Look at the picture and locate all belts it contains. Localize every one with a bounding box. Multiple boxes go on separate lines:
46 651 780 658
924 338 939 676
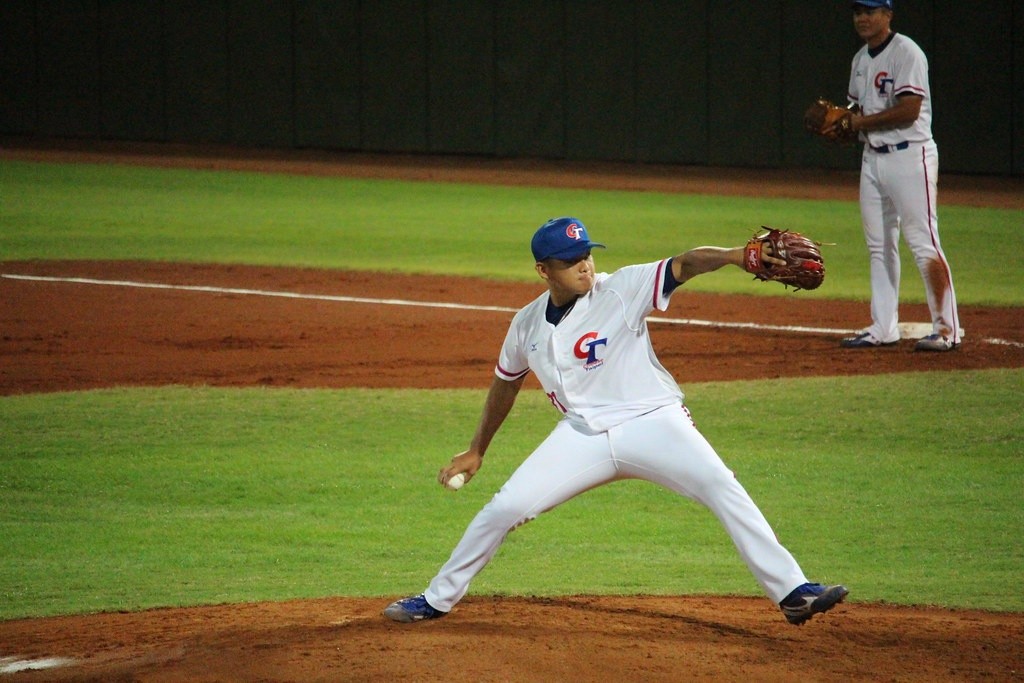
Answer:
869 141 908 154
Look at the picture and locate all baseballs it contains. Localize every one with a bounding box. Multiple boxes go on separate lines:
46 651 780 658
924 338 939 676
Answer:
444 472 465 490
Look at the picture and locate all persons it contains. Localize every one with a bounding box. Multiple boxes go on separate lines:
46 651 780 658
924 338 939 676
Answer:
383 217 849 626
803 0 962 352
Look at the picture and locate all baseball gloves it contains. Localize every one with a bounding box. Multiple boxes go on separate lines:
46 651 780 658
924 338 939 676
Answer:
803 95 859 150
742 222 839 293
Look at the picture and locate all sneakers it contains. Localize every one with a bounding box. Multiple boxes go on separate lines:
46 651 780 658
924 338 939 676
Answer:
780 581 848 626
917 333 960 351
841 332 897 347
385 594 447 623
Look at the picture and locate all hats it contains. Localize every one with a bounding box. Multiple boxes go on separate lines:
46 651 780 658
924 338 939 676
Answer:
532 216 606 262
853 0 892 10
